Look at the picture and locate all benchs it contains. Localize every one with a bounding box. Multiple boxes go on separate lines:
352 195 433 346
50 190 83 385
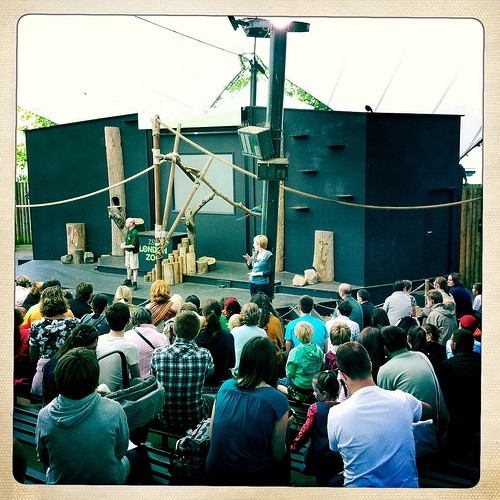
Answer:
12 386 472 488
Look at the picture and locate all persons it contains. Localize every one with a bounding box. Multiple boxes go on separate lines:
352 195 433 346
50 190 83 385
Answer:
206 336 290 486
242 234 275 302
34 347 132 485
276 273 482 460
120 218 140 291
14 273 285 435
327 341 432 488
290 370 345 475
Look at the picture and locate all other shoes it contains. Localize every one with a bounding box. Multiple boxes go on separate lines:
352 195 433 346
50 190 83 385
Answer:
132 283 137 291
120 279 132 287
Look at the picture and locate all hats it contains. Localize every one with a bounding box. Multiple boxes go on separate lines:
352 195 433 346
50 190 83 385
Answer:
458 315 478 333
223 297 241 317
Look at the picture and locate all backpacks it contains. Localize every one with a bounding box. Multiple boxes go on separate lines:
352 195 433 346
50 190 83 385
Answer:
167 416 212 485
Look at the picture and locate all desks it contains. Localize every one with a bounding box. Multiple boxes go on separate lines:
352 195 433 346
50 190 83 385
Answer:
137 229 187 271
